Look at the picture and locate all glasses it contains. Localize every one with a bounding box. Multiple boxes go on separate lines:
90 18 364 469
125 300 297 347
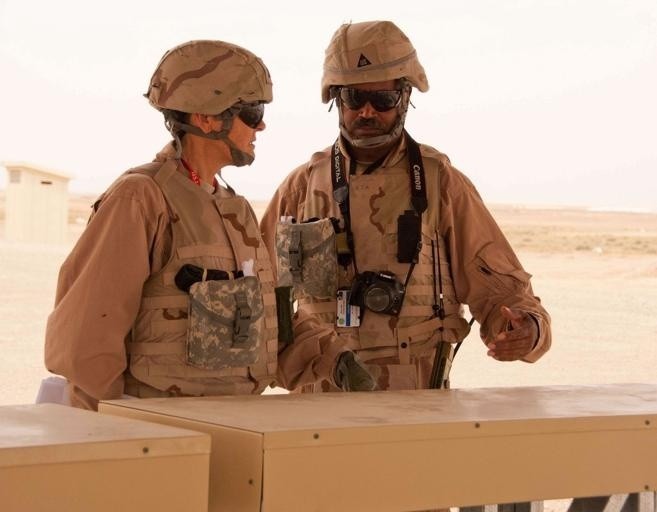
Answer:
340 87 402 111
238 104 264 128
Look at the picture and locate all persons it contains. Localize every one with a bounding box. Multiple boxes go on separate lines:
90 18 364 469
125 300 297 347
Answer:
261 19 552 394
43 40 382 413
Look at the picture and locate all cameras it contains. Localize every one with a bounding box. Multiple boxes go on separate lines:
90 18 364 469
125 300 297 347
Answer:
347 270 406 316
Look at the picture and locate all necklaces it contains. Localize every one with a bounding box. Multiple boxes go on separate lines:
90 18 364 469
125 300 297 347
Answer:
182 158 221 195
342 137 398 182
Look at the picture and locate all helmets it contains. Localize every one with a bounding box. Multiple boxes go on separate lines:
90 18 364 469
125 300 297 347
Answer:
322 22 428 104
147 40 272 115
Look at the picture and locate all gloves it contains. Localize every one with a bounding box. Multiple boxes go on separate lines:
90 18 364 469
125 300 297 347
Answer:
337 351 377 391
